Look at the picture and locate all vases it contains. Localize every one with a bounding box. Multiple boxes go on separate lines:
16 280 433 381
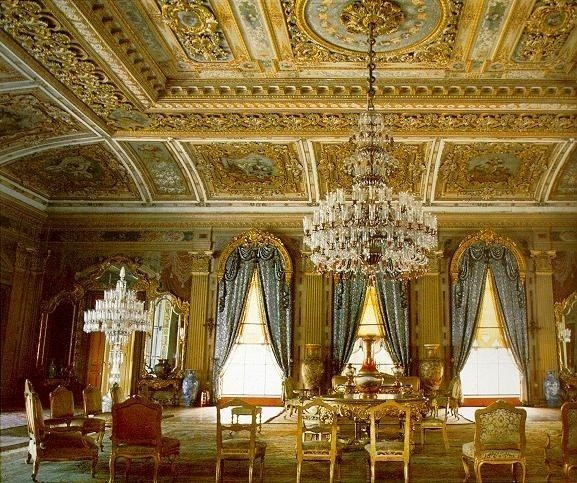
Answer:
163 358 172 375
301 342 324 395
419 343 444 397
46 358 58 381
182 368 199 408
543 371 561 408
154 359 168 379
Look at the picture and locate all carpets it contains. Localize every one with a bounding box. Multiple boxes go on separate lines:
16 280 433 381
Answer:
0 419 565 483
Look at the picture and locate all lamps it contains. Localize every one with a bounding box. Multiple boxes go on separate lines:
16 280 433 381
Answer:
83 267 155 410
299 0 440 282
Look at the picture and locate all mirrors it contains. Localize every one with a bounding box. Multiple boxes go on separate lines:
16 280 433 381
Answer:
139 292 190 389
36 291 82 385
554 291 577 387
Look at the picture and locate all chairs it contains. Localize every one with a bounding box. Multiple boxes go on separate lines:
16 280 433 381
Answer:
419 400 451 451
362 399 412 483
213 397 268 482
543 401 576 482
295 398 338 482
460 398 528 483
282 377 309 418
21 379 180 483
